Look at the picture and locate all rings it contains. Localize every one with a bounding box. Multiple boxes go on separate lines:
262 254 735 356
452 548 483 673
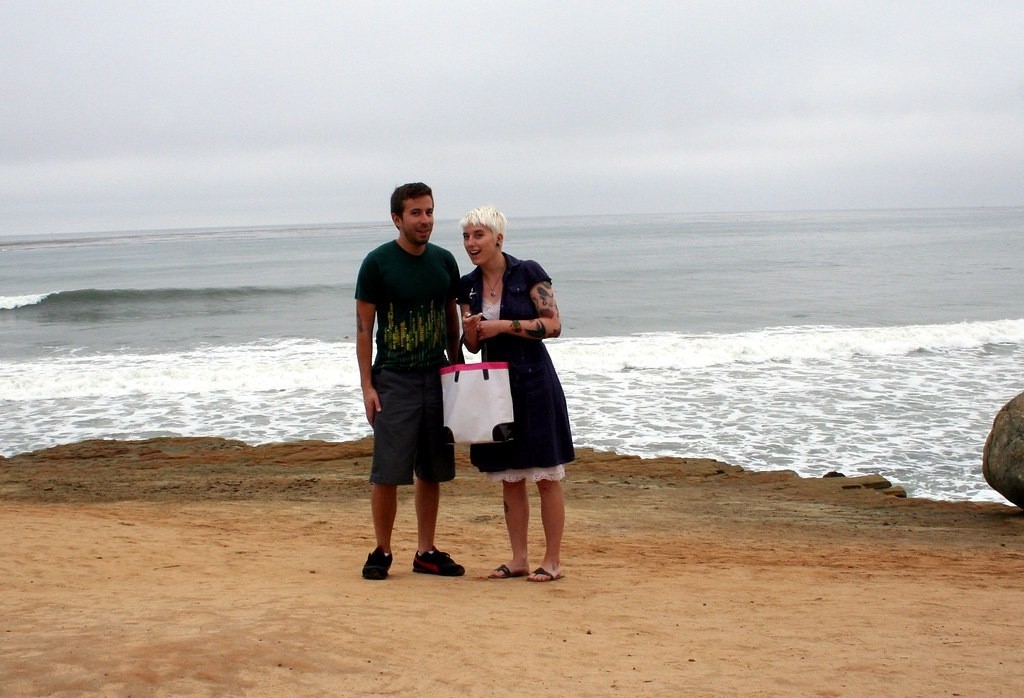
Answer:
476 327 481 331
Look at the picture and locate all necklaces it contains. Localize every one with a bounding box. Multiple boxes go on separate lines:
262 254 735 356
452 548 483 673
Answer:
481 269 504 297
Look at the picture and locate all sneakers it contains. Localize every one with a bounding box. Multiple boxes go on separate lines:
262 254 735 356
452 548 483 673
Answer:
362 545 392 579
413 546 465 576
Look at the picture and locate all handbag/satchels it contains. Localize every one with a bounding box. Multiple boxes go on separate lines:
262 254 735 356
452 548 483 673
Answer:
440 331 516 443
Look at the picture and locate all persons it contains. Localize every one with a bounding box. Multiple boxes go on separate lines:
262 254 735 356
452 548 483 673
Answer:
353 182 465 580
458 206 575 582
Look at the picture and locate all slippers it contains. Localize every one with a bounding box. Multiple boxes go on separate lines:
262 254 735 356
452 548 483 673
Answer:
528 568 565 581
488 564 530 579
